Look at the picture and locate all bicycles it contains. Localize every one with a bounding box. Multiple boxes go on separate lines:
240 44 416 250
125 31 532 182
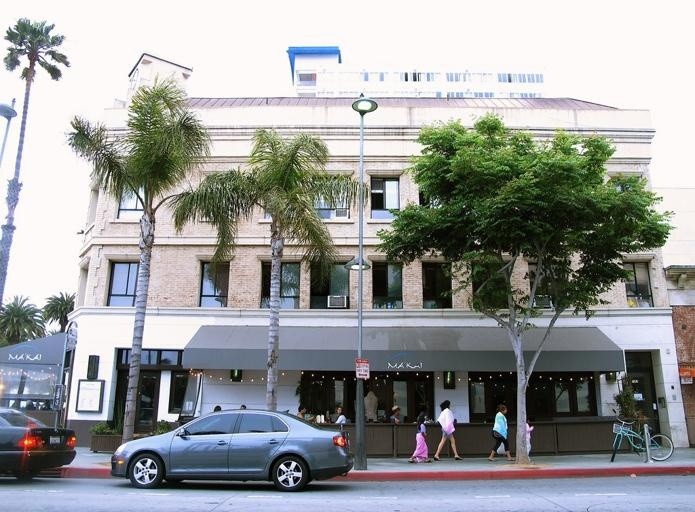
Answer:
611 408 674 462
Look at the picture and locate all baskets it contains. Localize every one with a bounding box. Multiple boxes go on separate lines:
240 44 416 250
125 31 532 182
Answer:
613 422 630 437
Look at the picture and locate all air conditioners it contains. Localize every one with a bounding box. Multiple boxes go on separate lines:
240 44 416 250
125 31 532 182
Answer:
327 295 349 309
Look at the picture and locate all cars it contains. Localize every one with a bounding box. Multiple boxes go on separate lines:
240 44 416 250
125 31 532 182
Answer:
0 407 76 482
111 408 353 492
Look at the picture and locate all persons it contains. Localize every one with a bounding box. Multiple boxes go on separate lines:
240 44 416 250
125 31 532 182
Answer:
214 406 221 411
432 399 463 460
488 404 515 461
239 404 246 409
297 405 316 422
525 416 536 457
334 405 346 423
389 405 401 423
408 411 431 463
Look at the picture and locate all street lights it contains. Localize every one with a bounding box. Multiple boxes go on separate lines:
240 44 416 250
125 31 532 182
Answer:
346 93 378 470
